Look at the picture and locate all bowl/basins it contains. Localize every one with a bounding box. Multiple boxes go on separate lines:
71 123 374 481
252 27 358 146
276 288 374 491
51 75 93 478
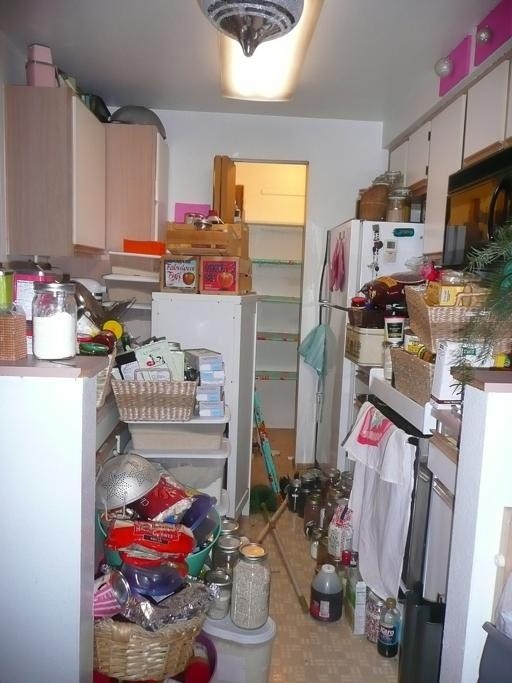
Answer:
97 505 223 580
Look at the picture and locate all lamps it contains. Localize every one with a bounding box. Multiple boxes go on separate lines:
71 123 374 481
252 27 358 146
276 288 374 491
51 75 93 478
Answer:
197 0 306 59
215 1 323 103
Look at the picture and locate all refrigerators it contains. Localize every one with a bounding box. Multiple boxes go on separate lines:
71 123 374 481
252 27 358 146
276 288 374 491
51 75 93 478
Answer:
313 221 424 486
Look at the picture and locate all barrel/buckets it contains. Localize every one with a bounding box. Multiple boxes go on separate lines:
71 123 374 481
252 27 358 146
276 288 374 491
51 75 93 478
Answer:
309 564 344 622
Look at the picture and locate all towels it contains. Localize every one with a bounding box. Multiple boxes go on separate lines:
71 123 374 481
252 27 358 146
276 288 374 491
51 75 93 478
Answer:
298 324 325 374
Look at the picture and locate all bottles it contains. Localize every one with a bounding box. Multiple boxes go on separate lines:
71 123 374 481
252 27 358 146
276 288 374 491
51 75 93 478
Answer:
168 341 181 352
93 328 117 353
376 597 399 657
342 548 361 607
181 640 210 683
383 303 435 363
0 263 14 313
207 517 272 630
373 168 410 222
284 467 354 558
30 280 77 361
350 296 366 308
184 208 221 230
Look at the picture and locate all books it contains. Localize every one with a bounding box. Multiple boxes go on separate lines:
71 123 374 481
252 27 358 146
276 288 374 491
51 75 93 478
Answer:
115 339 186 383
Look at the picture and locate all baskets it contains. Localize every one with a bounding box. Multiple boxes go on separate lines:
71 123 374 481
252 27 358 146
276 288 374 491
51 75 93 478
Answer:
110 371 202 423
95 348 120 409
92 613 207 682
389 344 437 408
403 276 512 356
0 313 28 362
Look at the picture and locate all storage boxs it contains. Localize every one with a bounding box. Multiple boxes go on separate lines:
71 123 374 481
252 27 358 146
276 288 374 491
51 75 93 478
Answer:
131 436 230 506
120 404 230 452
343 324 386 366
101 252 163 344
202 608 278 683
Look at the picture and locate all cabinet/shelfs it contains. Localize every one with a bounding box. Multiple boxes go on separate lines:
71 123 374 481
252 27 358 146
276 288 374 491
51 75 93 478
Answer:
389 47 512 194
250 223 305 431
1 84 106 257
448 168 506 246
0 338 137 682
102 123 171 256
